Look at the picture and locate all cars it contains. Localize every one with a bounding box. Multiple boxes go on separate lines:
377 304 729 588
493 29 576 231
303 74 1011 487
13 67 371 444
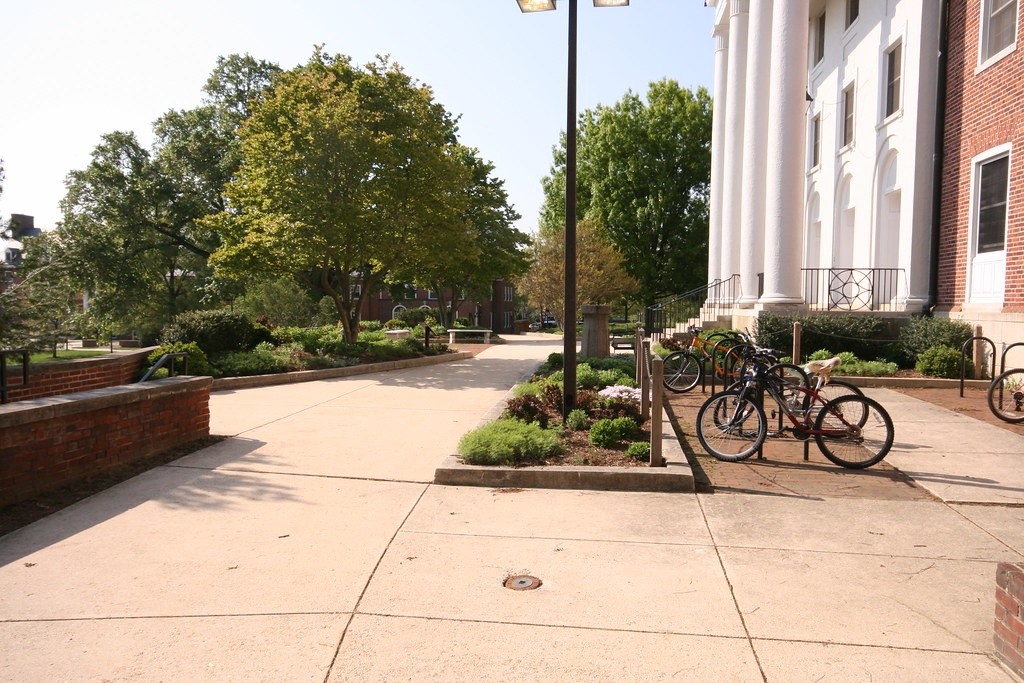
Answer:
529 315 558 332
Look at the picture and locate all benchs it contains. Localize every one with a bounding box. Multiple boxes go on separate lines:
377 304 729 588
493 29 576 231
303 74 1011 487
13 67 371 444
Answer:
385 330 410 339
448 328 493 343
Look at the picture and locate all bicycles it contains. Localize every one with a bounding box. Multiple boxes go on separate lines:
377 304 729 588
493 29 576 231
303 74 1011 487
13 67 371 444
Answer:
695 345 895 471
986 365 1024 424
662 323 870 438
